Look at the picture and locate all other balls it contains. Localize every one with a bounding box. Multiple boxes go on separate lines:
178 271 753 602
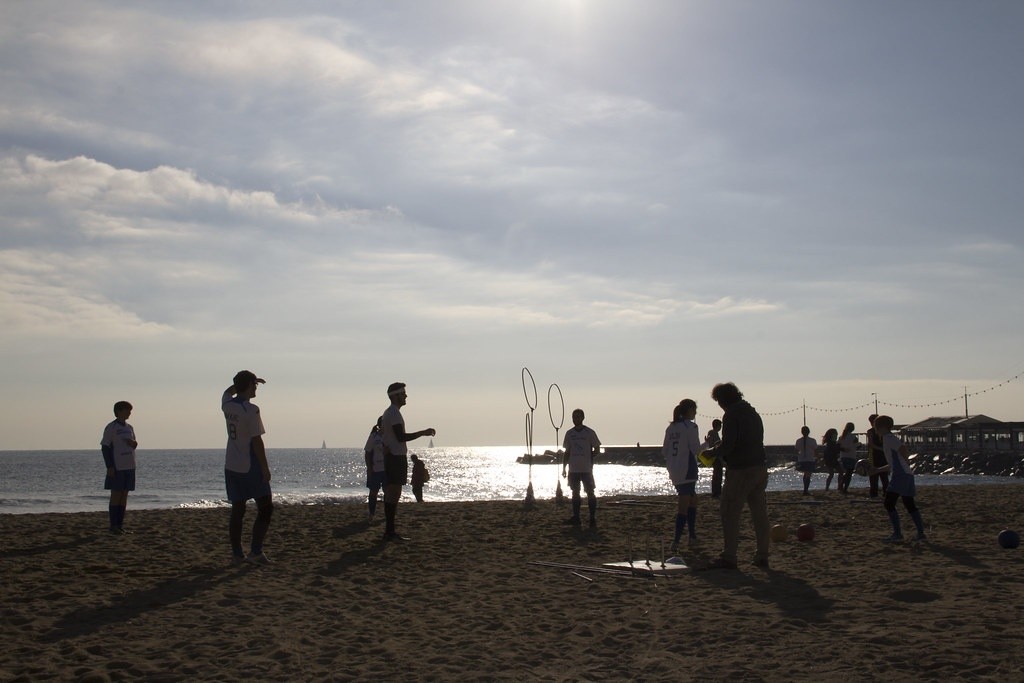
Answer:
998 530 1021 549
797 523 814 541
855 459 871 476
769 522 788 541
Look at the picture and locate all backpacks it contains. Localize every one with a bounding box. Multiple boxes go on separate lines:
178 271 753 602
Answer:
423 468 430 483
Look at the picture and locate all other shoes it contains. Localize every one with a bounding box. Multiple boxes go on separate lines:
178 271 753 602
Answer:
232 551 273 571
383 532 411 543
911 533 928 541
882 533 905 543
671 541 685 550
589 517 596 529
569 516 581 525
109 527 133 535
688 537 705 546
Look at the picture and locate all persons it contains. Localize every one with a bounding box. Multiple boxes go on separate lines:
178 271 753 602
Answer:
822 428 849 490
562 409 602 526
866 413 890 496
410 455 430 503
840 422 860 494
221 371 277 566
706 419 723 498
99 401 138 535
382 382 436 543
363 416 383 525
703 383 771 565
871 415 929 539
661 399 713 551
794 426 818 495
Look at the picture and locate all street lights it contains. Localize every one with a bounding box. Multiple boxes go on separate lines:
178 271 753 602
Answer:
871 392 878 415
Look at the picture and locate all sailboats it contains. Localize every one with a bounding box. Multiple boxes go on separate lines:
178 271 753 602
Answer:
428 439 434 448
321 440 326 449
